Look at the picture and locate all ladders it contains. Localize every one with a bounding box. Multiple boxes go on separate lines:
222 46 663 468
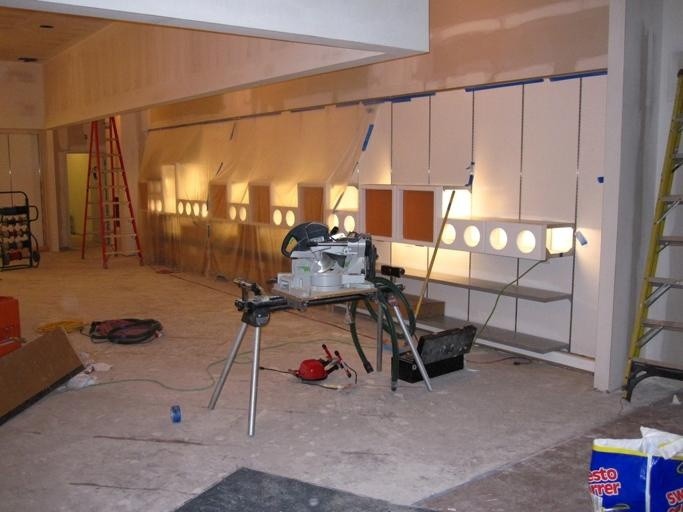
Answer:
621 68 683 403
81 114 145 270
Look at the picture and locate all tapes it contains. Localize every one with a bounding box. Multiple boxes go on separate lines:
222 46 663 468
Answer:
171 405 180 422
